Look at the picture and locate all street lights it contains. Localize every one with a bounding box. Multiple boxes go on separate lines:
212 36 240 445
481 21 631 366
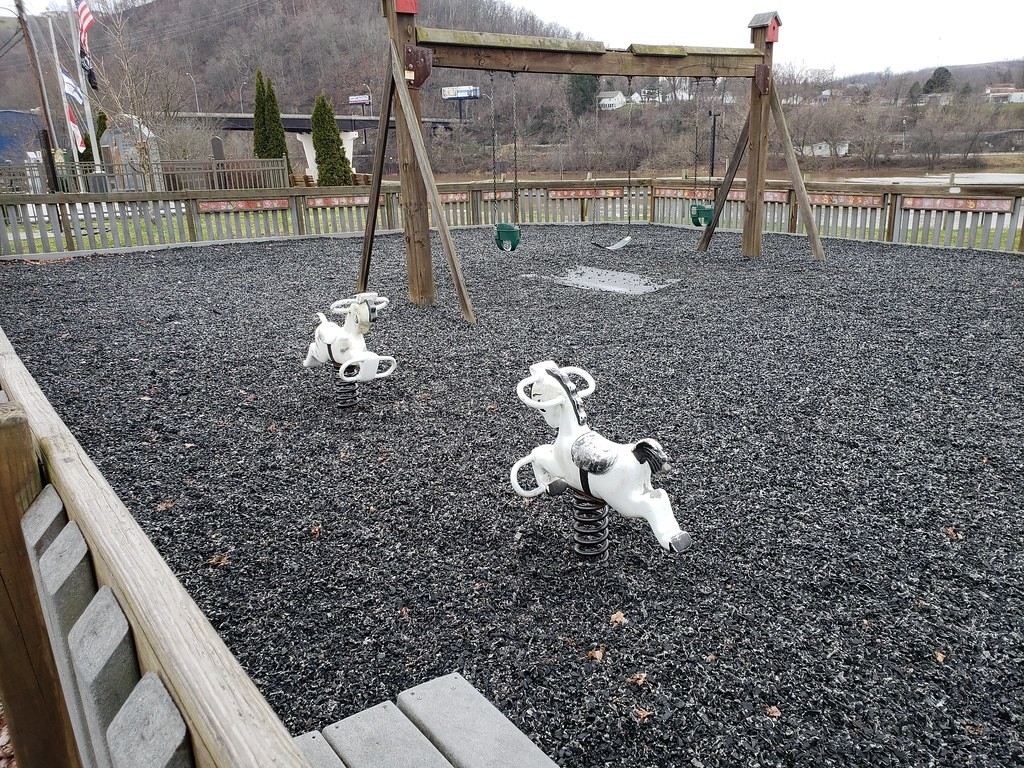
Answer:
363 84 372 116
185 73 199 112
240 82 247 114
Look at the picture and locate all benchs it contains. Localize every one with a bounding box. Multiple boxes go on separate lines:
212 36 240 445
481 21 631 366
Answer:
290 671 562 766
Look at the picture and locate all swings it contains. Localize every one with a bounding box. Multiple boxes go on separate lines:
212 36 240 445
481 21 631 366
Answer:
690 79 716 228
489 73 522 252
590 76 633 251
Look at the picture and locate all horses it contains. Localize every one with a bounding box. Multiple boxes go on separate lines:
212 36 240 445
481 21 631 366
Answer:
509 360 694 555
302 292 397 381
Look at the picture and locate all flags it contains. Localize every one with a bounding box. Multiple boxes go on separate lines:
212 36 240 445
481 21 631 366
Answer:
74 0 95 52
61 68 84 106
79 34 98 92
68 104 86 153
